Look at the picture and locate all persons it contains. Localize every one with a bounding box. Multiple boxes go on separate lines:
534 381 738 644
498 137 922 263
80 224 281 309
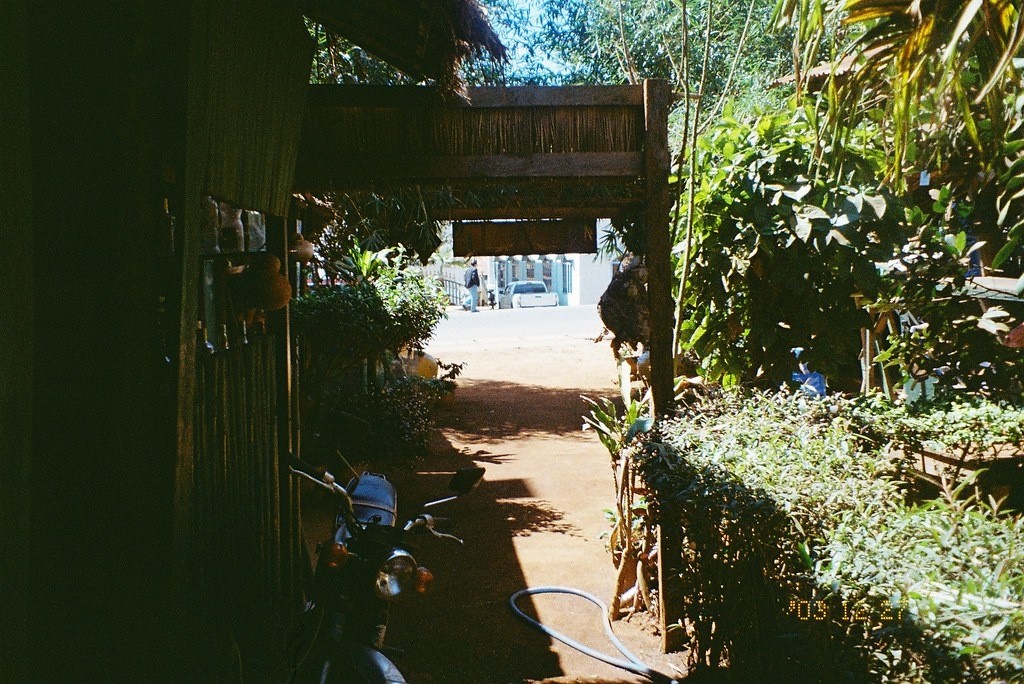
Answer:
462 259 480 312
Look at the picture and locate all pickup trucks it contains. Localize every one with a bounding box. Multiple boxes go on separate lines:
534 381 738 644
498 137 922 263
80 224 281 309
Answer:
498 281 558 308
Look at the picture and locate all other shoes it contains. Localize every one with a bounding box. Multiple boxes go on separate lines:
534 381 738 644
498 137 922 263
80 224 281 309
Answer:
461 304 468 311
471 308 479 312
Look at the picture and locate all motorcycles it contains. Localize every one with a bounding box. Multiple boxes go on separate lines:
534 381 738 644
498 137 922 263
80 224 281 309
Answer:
283 438 485 684
